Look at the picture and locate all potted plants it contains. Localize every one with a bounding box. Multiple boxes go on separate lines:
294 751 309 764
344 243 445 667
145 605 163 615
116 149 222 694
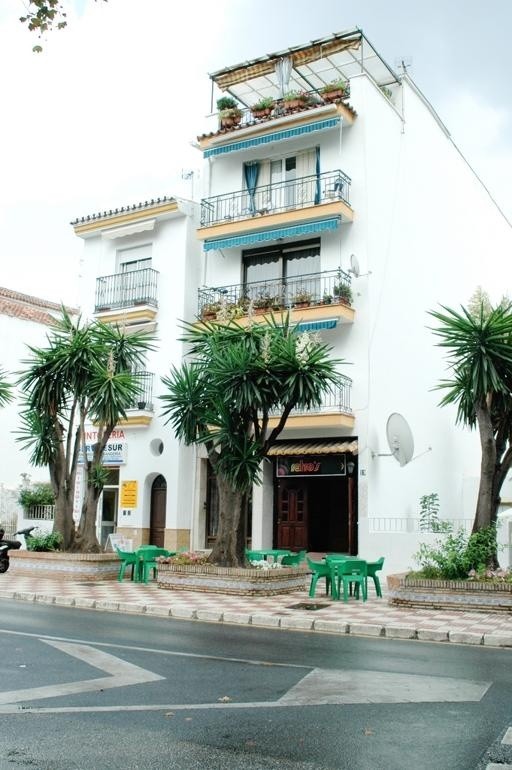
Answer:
217 78 348 128
200 281 351 320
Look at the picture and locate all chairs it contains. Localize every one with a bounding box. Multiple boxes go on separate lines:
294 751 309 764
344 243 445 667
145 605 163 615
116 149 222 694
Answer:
245 547 307 566
115 544 187 584
305 555 385 603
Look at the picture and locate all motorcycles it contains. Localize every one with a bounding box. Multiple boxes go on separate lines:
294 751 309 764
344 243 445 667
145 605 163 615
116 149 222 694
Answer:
0 526 39 573
